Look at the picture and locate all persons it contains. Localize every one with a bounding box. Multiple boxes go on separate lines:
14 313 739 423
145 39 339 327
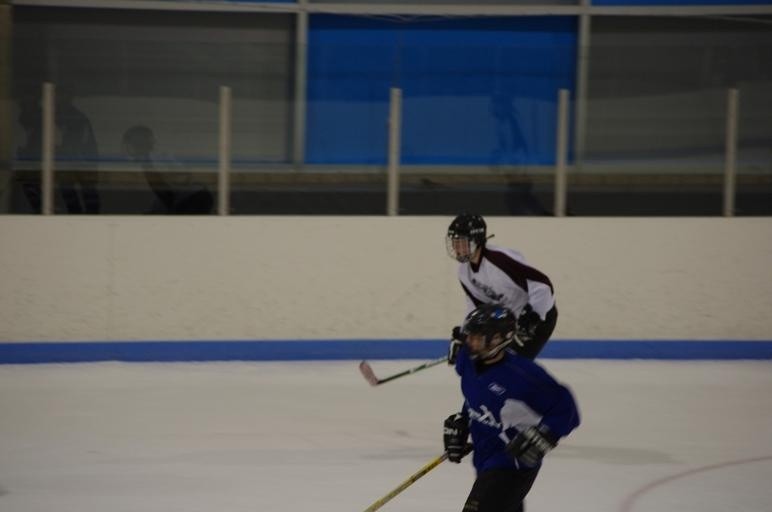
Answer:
445 211 558 366
442 304 581 512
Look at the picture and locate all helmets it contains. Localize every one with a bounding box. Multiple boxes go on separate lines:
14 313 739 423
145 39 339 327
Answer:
445 212 486 262
461 305 515 362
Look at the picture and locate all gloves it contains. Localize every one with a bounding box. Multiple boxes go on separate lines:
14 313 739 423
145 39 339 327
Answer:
443 412 467 463
509 428 552 471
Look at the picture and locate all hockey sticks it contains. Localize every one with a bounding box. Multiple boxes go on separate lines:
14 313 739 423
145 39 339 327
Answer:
360 357 449 385
